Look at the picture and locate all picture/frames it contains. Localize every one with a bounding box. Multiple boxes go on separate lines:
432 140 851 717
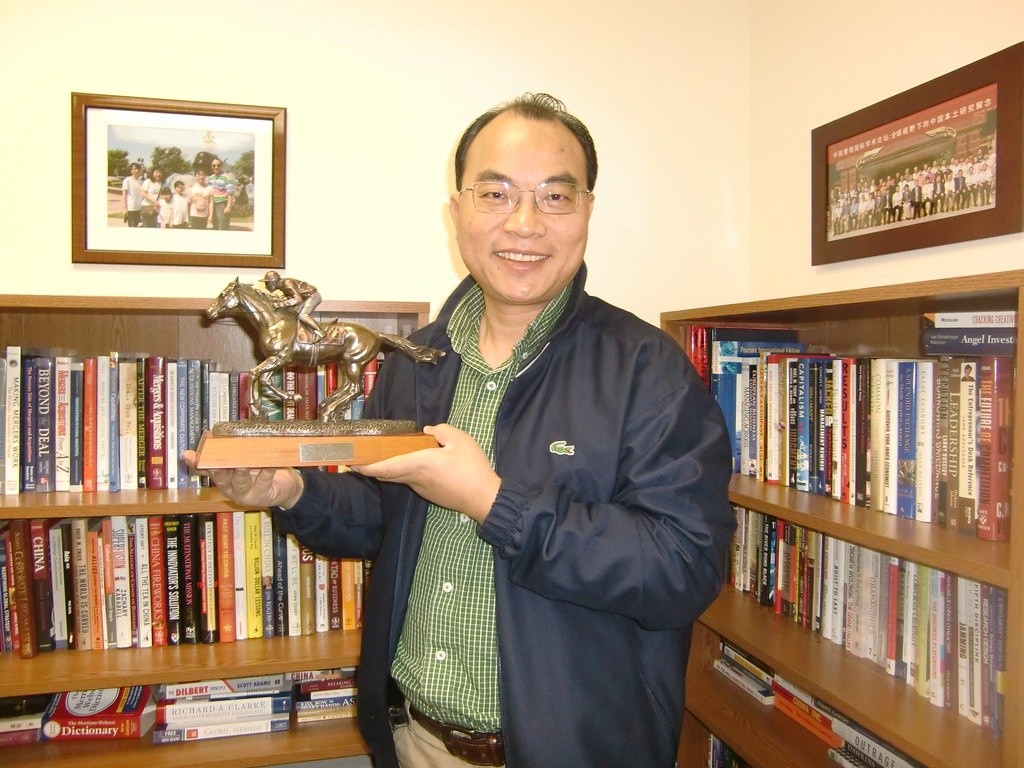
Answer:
71 91 288 268
810 40 1024 266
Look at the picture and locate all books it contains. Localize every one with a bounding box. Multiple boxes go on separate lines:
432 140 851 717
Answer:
707 734 750 768
0 510 374 659
714 640 924 768
728 505 1007 739
0 346 385 492
685 311 1019 541
0 667 358 747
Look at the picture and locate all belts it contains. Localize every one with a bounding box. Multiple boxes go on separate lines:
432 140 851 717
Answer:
402 699 505 767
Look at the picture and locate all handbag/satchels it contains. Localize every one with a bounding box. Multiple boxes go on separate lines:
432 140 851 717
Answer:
153 206 160 214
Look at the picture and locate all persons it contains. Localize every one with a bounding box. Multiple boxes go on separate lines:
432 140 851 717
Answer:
961 364 975 382
828 145 997 236
263 576 272 590
122 158 254 230
182 92 737 768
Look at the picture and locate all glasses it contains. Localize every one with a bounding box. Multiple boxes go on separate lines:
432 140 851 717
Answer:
212 165 220 168
459 181 589 214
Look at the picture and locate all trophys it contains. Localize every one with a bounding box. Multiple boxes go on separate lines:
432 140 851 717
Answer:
196 271 446 468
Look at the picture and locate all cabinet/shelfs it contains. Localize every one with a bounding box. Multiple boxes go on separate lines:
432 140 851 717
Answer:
0 295 432 768
658 267 1023 768
